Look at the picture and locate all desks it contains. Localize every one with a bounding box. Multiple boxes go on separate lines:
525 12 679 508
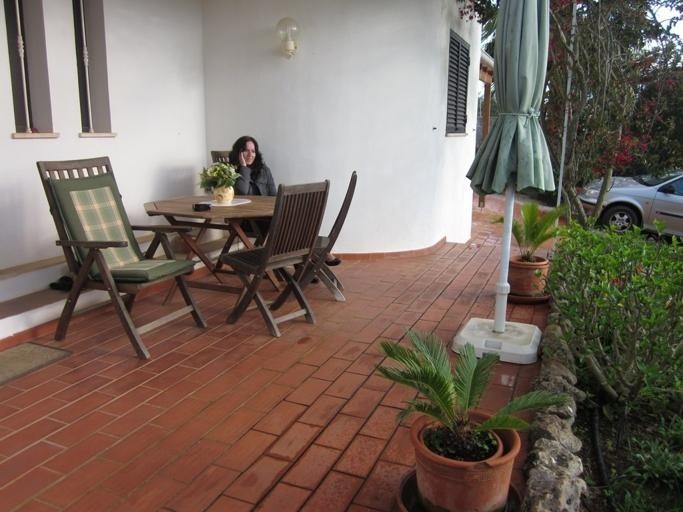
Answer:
143 195 283 313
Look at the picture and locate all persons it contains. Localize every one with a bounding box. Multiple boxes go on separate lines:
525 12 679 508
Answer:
229 136 342 284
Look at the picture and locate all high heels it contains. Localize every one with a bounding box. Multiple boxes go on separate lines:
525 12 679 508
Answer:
292 252 341 284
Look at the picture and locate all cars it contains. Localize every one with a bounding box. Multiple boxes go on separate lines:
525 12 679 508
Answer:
575 169 682 246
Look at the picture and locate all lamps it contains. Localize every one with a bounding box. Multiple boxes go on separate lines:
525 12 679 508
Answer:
276 16 301 58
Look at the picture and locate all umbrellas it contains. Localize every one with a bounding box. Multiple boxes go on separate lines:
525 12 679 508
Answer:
466 0 556 333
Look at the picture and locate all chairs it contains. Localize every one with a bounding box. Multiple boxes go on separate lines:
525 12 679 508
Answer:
36 156 209 359
287 171 357 301
220 179 330 338
211 151 285 281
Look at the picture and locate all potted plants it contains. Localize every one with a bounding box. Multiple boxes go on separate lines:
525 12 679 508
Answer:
196 161 241 202
490 200 568 303
375 326 568 512
